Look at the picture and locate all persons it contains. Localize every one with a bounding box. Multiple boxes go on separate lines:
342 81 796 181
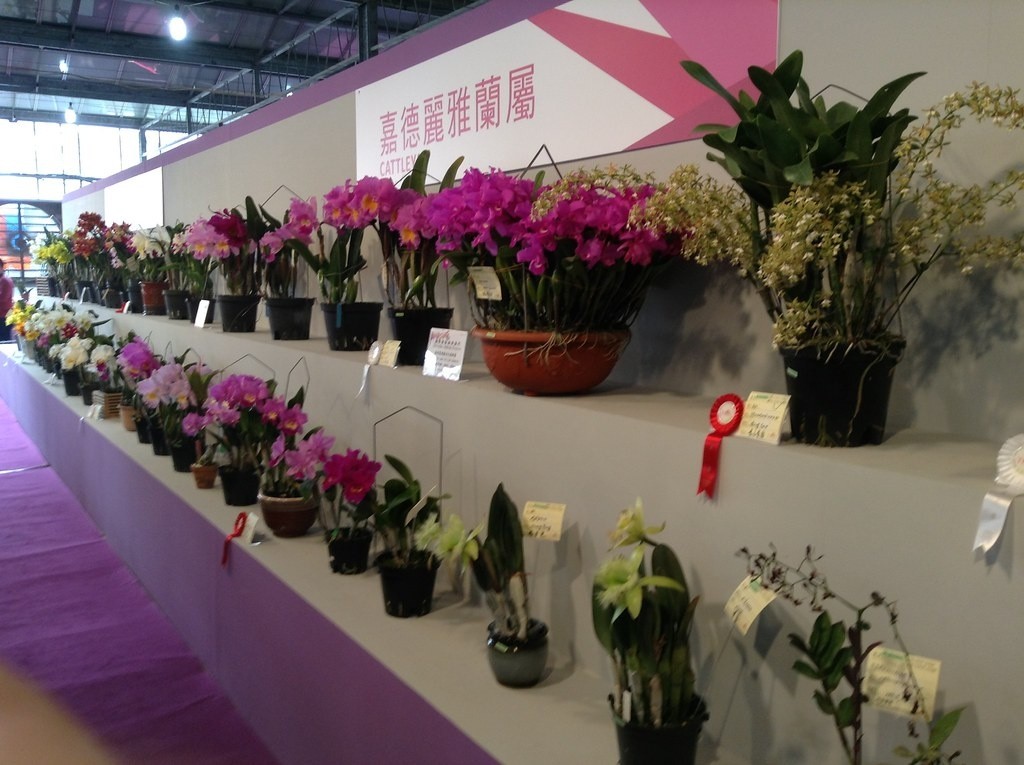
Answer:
0 259 13 341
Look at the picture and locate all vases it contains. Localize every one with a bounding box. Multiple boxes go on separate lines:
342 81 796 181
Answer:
185 298 216 324
15 335 22 351
471 325 632 396
79 382 101 405
327 527 372 574
18 334 27 352
321 302 383 353
138 282 170 316
388 307 454 366
53 361 63 379
98 281 121 306
778 330 906 448
192 463 218 489
56 283 62 297
75 281 93 299
24 339 37 358
116 403 142 431
132 414 161 443
45 359 55 373
149 423 175 456
218 464 264 506
487 618 549 688
218 295 262 333
257 480 319 538
170 441 200 471
376 550 440 618
265 298 316 342
87 287 97 303
36 276 55 296
162 290 190 319
38 351 43 365
62 370 81 395
611 693 709 764
126 287 143 313
92 389 123 419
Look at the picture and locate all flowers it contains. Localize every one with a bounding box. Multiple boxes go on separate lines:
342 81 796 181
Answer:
33 50 1024 449
5 289 967 764
